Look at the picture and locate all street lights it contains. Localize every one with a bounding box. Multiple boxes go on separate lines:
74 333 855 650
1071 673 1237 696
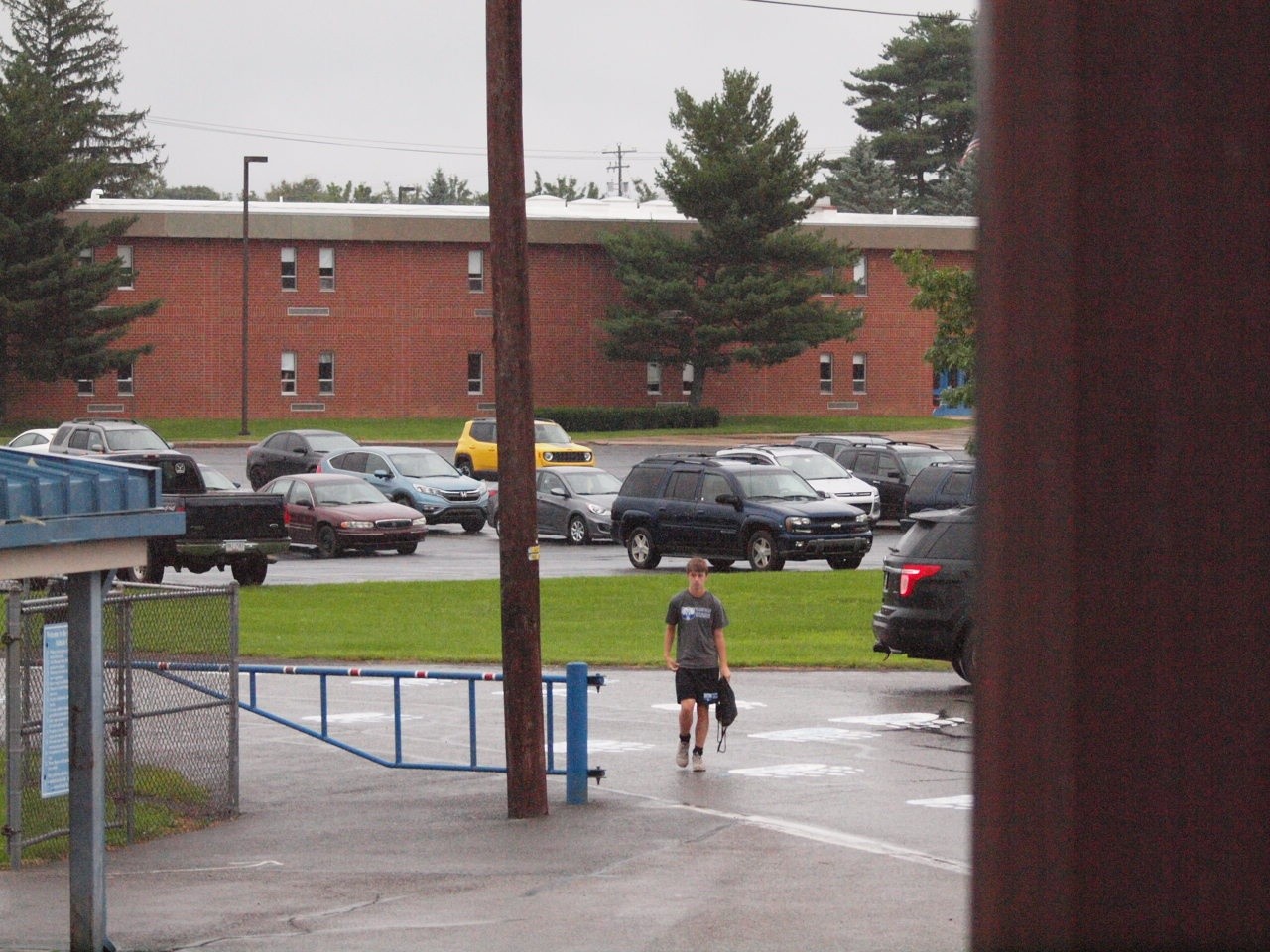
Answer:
240 154 268 435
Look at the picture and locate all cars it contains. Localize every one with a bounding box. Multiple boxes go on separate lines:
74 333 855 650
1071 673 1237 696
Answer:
714 443 882 523
196 463 242 493
484 464 623 546
254 472 429 559
6 427 57 455
245 429 362 493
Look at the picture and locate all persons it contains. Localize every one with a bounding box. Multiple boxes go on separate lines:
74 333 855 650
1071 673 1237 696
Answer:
664 557 731 771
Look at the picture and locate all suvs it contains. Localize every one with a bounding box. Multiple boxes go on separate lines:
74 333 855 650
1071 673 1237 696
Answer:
898 459 977 535
453 415 596 481
833 440 960 533
870 503 978 684
315 445 491 534
609 451 873 573
48 416 175 456
789 433 898 477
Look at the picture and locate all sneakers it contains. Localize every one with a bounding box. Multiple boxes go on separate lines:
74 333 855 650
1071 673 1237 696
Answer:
675 737 690 767
691 751 707 771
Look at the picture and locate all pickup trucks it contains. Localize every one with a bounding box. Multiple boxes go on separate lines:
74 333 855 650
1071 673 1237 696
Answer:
72 449 293 586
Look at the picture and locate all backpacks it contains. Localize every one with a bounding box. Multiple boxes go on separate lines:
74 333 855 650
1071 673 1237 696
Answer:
716 676 738 753
